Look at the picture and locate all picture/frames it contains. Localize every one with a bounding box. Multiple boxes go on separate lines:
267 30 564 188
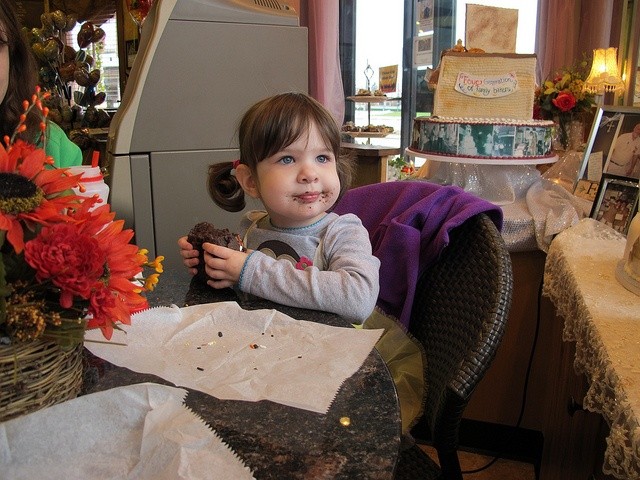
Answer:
571 178 600 204
574 104 640 180
588 173 640 236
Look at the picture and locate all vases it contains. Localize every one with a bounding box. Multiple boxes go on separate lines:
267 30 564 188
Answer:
0 316 163 422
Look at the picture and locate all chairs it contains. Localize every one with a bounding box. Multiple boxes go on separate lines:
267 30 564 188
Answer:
332 179 515 480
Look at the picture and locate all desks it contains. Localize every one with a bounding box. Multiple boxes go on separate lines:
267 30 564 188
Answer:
542 216 640 480
83 275 401 480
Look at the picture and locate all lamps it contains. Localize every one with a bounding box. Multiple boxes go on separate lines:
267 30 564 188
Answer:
581 47 624 105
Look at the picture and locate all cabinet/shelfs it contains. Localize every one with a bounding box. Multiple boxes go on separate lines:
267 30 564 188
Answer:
539 309 603 480
462 252 556 432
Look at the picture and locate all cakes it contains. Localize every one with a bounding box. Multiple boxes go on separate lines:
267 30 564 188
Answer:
410 116 555 159
184 223 246 284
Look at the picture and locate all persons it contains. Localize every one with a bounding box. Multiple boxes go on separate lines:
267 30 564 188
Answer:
0 3 86 224
177 94 381 327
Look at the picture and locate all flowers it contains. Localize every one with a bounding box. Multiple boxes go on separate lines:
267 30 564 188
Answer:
0 85 166 340
535 61 596 147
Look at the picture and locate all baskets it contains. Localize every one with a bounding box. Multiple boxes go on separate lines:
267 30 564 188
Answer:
0 311 84 424
